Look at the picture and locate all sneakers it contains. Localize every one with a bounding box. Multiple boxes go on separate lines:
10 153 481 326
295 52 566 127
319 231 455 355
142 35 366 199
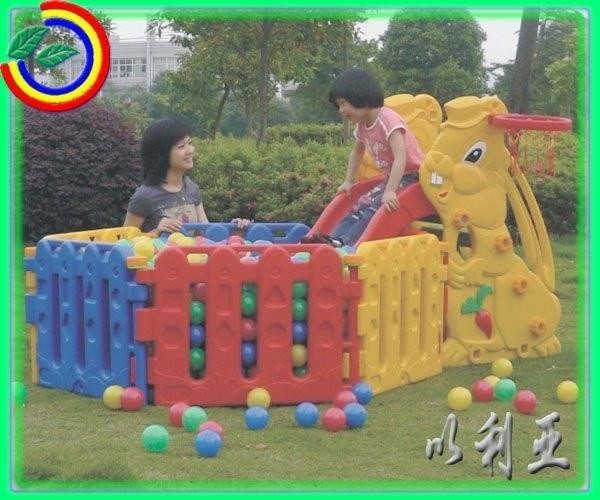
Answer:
301 237 324 243
315 233 345 248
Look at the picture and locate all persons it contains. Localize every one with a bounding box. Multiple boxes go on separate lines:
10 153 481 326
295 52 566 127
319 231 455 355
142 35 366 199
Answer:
300 67 426 248
123 120 254 238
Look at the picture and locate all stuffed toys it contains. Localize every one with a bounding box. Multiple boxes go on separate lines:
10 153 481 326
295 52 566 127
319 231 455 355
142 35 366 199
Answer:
103 232 373 457
447 358 579 415
